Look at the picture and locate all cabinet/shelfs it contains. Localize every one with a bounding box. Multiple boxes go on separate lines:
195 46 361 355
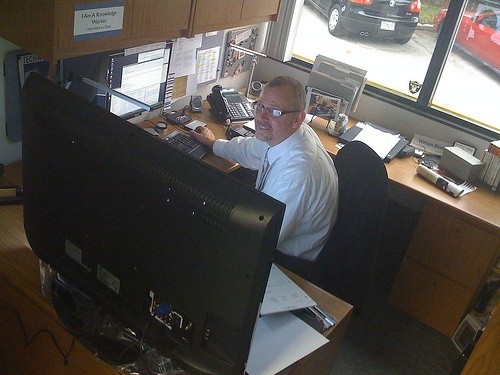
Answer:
0 0 500 375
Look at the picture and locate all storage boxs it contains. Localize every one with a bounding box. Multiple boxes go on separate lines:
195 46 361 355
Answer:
439 146 483 182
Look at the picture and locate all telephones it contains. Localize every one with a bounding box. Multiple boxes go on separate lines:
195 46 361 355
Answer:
207 84 255 121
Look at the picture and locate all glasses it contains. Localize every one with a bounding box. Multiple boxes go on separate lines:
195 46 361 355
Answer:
255 103 297 117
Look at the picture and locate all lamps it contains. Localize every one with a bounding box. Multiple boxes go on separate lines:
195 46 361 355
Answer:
63 73 153 112
227 42 267 104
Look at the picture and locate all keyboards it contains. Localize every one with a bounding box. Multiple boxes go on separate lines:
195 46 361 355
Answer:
166 132 208 159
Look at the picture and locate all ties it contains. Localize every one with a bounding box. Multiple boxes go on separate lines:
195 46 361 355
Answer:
255 150 270 189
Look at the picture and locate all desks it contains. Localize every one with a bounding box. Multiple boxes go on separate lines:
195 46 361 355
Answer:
0 163 353 375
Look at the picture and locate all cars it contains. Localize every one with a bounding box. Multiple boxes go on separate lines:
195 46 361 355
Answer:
304 0 422 45
433 9 500 75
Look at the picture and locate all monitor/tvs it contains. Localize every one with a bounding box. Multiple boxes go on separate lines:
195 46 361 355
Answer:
20 71 285 375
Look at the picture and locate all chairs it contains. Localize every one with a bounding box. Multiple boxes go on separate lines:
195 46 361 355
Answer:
281 142 387 316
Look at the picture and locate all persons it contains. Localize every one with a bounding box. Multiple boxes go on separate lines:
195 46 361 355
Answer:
189 76 338 280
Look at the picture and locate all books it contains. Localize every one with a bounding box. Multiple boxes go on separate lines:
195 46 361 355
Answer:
415 163 478 199
479 140 500 192
